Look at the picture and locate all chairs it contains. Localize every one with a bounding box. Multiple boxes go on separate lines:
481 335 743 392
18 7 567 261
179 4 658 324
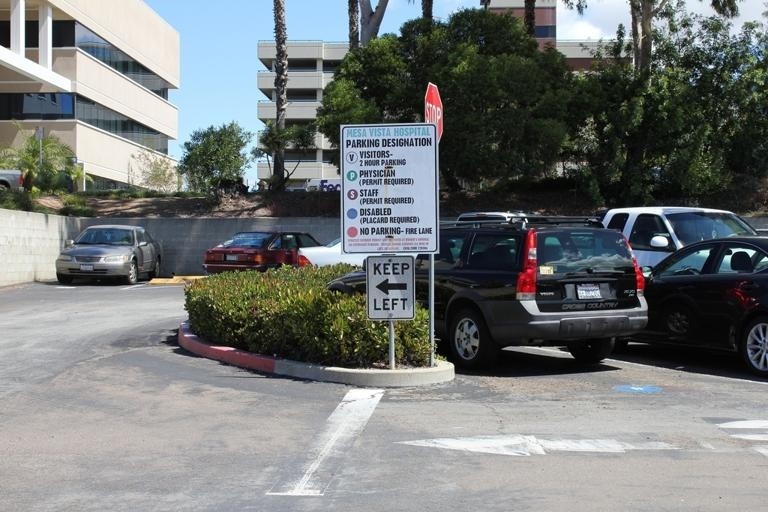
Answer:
730 251 753 273
421 242 565 272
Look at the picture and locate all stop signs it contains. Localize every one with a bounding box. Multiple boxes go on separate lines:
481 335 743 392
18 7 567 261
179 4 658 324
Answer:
424 82 444 145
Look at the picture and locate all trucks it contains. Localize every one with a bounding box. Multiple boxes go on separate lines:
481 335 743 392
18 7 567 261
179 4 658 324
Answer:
305 178 341 192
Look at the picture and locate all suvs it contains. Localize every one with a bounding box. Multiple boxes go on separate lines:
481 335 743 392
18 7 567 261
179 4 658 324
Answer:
326 216 649 364
601 207 768 274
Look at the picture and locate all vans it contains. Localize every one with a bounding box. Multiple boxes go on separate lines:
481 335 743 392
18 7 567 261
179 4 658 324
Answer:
455 210 541 226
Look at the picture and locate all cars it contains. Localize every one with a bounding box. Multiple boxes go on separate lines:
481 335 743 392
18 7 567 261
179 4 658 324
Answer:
1 169 23 192
55 224 163 284
297 238 418 269
203 231 320 272
613 238 768 378
